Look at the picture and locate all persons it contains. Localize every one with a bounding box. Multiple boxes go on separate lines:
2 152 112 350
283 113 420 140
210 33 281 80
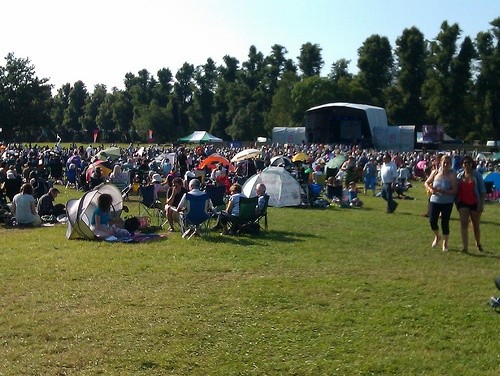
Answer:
37 187 66 224
237 183 268 234
165 177 188 232
424 154 458 251
177 179 216 238
12 183 39 227
454 158 486 253
0 133 500 217
90 193 140 239
217 183 249 237
0 193 12 223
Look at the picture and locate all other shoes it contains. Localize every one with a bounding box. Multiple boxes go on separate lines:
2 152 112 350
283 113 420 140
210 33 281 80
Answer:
168 227 174 232
443 243 448 252
431 237 441 247
476 244 483 251
461 248 467 253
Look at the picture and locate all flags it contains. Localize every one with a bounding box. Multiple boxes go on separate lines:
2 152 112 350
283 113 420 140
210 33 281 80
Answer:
148 129 153 142
93 129 98 143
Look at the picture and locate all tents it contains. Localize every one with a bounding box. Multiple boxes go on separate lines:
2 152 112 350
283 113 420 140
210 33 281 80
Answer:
242 166 307 209
179 131 224 146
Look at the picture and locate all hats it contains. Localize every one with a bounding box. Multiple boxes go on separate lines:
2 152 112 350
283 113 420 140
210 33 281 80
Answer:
9 165 16 169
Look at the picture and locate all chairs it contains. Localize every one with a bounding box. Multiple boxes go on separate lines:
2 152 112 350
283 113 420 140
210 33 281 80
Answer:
298 172 309 184
253 195 270 231
115 182 127 199
326 167 338 180
316 175 327 185
219 196 258 235
187 176 202 191
138 184 165 219
484 181 497 202
327 184 343 205
300 183 322 207
179 191 212 242
5 179 21 202
65 163 80 192
206 185 226 211
90 176 105 189
51 161 63 179
